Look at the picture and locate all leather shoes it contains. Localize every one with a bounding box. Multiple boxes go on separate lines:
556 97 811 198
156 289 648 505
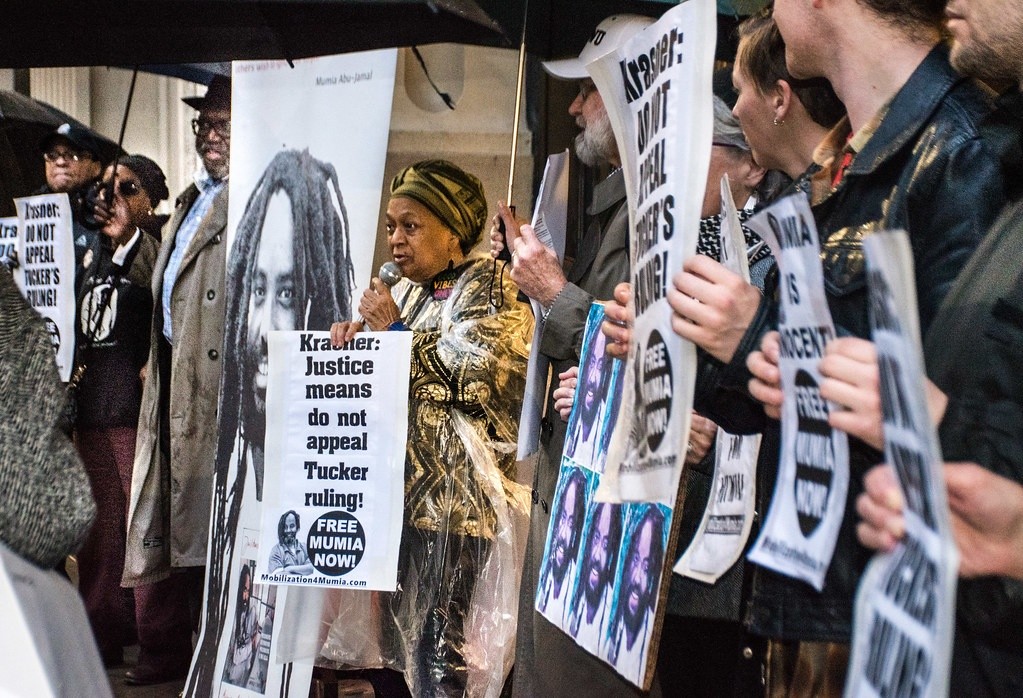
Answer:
124 658 190 682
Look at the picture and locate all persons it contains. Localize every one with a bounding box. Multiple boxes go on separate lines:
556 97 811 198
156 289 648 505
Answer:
490 0 1023 698
563 311 619 467
0 74 230 698
602 502 666 683
565 502 622 655
536 468 587 629
180 149 354 698
327 156 534 698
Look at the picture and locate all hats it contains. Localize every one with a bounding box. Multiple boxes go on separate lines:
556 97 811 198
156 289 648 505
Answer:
42 123 96 158
540 12 658 80
390 159 489 254
110 153 168 209
182 75 231 111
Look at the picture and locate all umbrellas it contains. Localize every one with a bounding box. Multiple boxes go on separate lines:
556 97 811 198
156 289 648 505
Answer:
0 0 769 214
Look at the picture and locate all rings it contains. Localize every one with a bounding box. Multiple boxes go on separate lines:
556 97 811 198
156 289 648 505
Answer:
687 443 692 453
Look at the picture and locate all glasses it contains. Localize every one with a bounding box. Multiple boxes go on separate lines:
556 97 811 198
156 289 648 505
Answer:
41 149 94 162
192 118 231 137
117 180 149 208
578 80 594 99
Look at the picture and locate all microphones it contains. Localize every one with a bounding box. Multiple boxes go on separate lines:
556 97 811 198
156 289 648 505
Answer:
357 261 404 325
